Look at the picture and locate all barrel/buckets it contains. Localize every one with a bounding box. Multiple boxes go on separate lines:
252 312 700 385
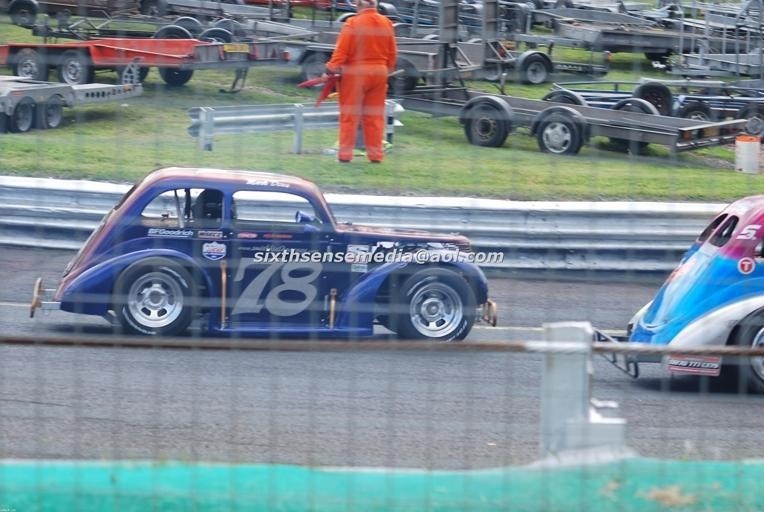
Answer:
735 136 760 173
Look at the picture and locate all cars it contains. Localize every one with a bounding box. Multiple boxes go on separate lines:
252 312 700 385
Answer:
593 193 764 393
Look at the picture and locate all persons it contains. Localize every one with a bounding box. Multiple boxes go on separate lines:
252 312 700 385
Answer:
322 0 397 164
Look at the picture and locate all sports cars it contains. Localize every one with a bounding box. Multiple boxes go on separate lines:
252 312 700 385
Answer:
30 165 500 344
